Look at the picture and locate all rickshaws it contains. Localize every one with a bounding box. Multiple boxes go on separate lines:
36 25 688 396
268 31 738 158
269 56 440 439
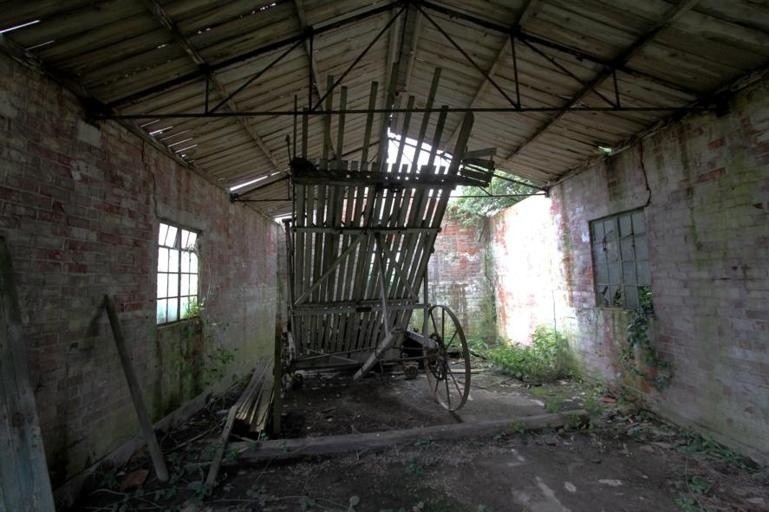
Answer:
267 66 500 418
